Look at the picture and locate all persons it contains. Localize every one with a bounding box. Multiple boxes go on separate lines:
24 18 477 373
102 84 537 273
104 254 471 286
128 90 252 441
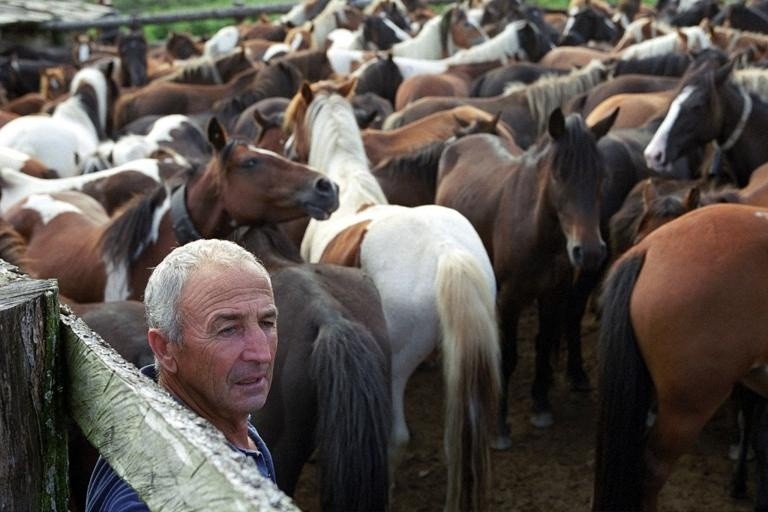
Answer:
81 238 278 512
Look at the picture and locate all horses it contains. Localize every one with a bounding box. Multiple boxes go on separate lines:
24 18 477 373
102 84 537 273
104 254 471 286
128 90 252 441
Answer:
0 0 768 511
